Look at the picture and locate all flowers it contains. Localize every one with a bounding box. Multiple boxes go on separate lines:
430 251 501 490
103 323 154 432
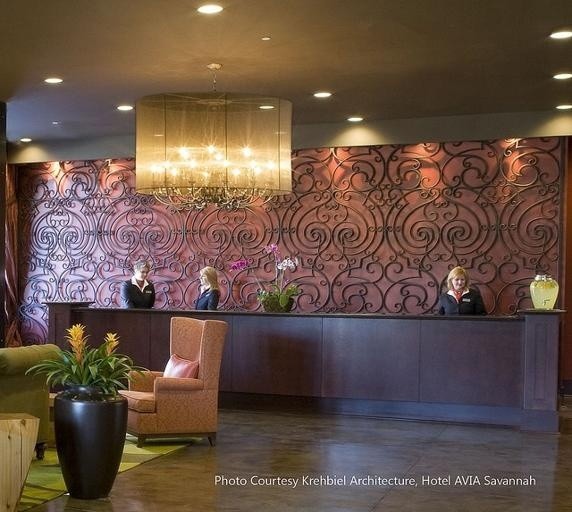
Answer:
227 242 302 308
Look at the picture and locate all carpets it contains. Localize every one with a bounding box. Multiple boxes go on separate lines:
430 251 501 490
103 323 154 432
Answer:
13 431 207 512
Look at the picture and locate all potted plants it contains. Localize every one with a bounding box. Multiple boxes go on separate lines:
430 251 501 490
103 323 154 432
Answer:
24 323 155 399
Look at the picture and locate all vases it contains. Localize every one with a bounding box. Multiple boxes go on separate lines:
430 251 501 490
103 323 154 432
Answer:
54 392 129 499
530 273 559 311
259 293 294 313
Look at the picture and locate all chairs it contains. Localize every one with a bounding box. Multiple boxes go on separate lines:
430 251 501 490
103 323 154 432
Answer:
116 316 228 448
0 343 65 460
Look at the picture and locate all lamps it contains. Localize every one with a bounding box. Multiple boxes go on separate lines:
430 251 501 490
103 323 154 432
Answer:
135 63 294 212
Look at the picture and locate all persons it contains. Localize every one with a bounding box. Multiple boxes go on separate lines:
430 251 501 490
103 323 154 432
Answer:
432 265 487 315
194 266 221 310
122 259 155 308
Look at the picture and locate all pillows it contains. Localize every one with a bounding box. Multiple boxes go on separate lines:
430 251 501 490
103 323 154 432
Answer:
162 354 199 380
